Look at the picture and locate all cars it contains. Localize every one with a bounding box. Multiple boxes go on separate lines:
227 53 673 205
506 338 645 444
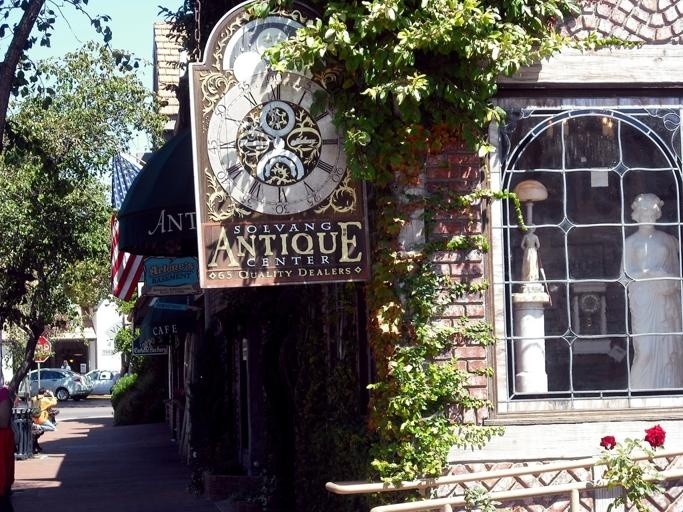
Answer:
16 369 119 401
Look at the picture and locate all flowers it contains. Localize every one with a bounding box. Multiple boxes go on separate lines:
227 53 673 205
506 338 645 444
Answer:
594 423 667 512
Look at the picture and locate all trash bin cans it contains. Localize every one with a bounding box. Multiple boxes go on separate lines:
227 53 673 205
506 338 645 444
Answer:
11 408 33 460
80 364 86 374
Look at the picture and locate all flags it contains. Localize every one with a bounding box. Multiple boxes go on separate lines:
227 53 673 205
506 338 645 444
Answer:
108 153 146 303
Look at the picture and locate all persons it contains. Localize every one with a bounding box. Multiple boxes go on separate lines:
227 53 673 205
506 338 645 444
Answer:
61 360 71 371
29 388 57 431
622 194 683 391
522 223 540 282
